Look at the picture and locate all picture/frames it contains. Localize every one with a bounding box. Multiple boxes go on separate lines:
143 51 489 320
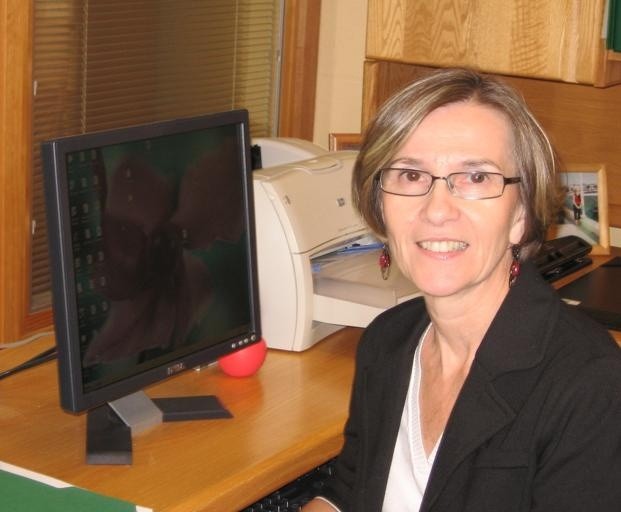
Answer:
544 161 612 259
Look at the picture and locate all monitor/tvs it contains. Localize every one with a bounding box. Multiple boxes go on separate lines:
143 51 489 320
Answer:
40 108 262 466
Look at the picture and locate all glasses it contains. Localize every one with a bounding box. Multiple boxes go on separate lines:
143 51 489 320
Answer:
376 167 522 200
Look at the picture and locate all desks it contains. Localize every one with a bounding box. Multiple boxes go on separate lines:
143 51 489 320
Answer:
2 249 620 512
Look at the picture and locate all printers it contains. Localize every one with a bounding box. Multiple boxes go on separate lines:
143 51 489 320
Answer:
253 135 423 353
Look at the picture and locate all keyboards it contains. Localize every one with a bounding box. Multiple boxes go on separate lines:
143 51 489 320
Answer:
240 459 337 511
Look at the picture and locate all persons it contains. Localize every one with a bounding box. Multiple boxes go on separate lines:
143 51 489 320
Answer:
570 187 582 226
298 66 621 512
